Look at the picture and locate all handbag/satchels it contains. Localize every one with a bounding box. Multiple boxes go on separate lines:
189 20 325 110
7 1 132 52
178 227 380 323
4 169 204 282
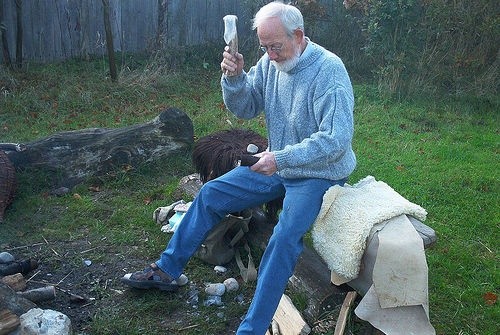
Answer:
193 206 256 282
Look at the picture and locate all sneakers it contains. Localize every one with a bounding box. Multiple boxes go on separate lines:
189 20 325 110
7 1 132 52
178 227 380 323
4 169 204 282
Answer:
121 263 180 292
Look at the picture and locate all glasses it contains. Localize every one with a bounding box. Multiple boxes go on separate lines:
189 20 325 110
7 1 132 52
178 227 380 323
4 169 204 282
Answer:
260 28 299 52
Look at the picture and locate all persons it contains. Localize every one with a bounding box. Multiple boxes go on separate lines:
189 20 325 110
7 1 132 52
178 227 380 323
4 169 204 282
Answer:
118 0 357 335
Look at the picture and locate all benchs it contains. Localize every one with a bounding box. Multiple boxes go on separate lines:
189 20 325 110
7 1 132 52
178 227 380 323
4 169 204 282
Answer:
192 128 438 335
